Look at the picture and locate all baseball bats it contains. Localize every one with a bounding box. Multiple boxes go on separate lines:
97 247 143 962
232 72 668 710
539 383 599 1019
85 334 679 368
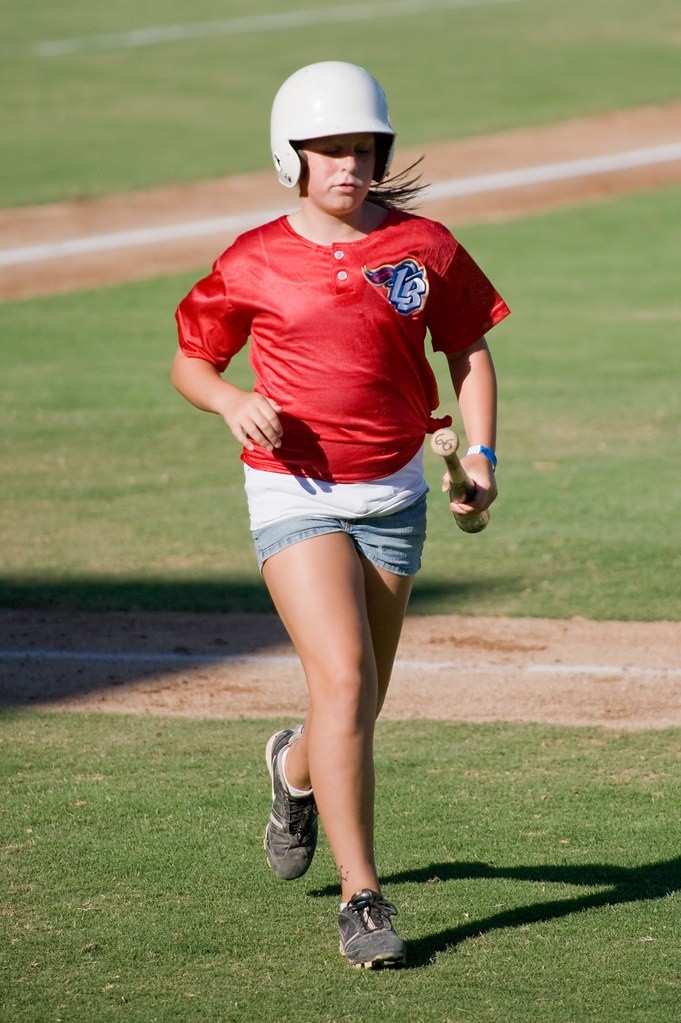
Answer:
430 427 491 534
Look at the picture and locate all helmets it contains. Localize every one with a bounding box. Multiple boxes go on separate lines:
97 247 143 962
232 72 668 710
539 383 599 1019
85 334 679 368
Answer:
270 60 397 189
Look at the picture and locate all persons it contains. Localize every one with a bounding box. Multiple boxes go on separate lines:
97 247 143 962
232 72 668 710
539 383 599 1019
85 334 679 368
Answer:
466 444 497 473
174 62 514 968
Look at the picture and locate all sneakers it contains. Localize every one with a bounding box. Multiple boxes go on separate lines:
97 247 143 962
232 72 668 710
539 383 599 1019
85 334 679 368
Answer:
263 729 319 881
336 888 407 970
